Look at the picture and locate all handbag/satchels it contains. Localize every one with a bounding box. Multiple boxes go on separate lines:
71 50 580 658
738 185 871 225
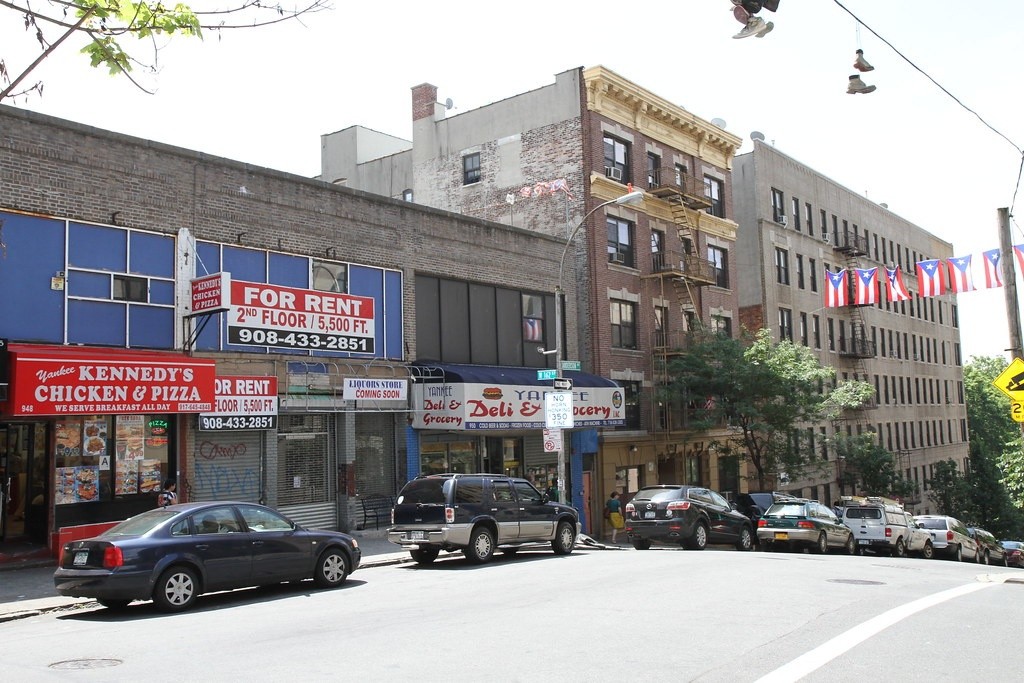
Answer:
603 499 612 519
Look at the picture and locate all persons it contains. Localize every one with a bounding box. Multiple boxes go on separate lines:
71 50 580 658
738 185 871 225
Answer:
158 479 179 507
744 501 761 545
201 514 217 534
604 491 624 544
545 478 561 503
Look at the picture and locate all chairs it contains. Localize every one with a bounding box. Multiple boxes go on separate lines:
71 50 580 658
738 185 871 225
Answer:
200 516 218 533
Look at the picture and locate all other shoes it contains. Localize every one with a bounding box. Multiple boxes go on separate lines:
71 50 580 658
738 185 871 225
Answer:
12 514 25 521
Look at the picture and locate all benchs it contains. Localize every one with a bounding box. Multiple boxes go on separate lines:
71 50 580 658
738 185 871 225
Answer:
361 496 395 530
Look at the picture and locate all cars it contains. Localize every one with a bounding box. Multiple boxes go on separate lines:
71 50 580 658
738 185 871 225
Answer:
1000 541 1024 568
53 500 362 615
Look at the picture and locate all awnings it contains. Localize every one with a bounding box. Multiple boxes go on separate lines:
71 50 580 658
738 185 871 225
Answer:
409 360 627 430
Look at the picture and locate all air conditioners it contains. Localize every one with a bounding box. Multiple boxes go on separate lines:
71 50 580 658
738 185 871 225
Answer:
778 216 788 224
609 253 624 263
606 167 622 180
891 350 898 357
823 233 830 241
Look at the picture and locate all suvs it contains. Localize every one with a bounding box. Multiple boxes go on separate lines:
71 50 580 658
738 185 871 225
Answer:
385 471 583 564
735 490 797 525
912 515 979 564
624 484 753 552
965 528 1008 567
756 497 855 555
841 501 934 558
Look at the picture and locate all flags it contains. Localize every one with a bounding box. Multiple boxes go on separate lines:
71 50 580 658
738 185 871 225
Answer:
822 242 1024 309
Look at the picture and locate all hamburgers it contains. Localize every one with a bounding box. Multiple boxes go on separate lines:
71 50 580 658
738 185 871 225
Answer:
115 462 137 493
116 429 143 451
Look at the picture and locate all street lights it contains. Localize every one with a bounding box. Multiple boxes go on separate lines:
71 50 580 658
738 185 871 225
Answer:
554 191 645 505
